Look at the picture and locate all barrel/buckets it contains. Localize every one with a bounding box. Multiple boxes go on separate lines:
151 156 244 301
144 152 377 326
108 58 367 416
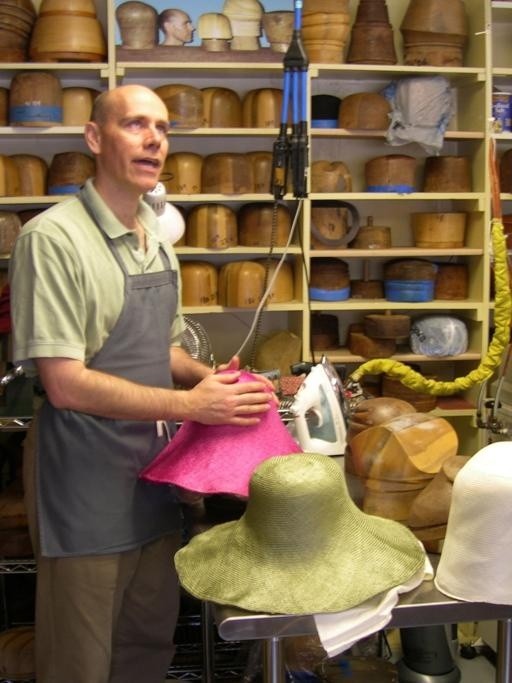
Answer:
409 211 467 248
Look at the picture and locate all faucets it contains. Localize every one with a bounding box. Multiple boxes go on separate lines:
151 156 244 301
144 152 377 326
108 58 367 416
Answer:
1 365 24 386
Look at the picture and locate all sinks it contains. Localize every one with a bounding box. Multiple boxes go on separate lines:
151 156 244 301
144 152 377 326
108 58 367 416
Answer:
0 417 32 428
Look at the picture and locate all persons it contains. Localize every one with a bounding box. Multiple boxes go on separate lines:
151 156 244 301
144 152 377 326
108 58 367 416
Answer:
8 84 281 682
159 9 196 46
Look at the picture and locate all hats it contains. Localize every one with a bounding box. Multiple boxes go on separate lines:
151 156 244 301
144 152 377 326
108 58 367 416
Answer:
137 369 306 498
173 453 425 617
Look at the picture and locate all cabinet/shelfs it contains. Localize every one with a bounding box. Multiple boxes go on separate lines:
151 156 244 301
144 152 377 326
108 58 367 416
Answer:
2 0 512 561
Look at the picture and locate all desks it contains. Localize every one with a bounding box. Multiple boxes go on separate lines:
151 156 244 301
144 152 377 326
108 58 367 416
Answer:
209 554 511 683
